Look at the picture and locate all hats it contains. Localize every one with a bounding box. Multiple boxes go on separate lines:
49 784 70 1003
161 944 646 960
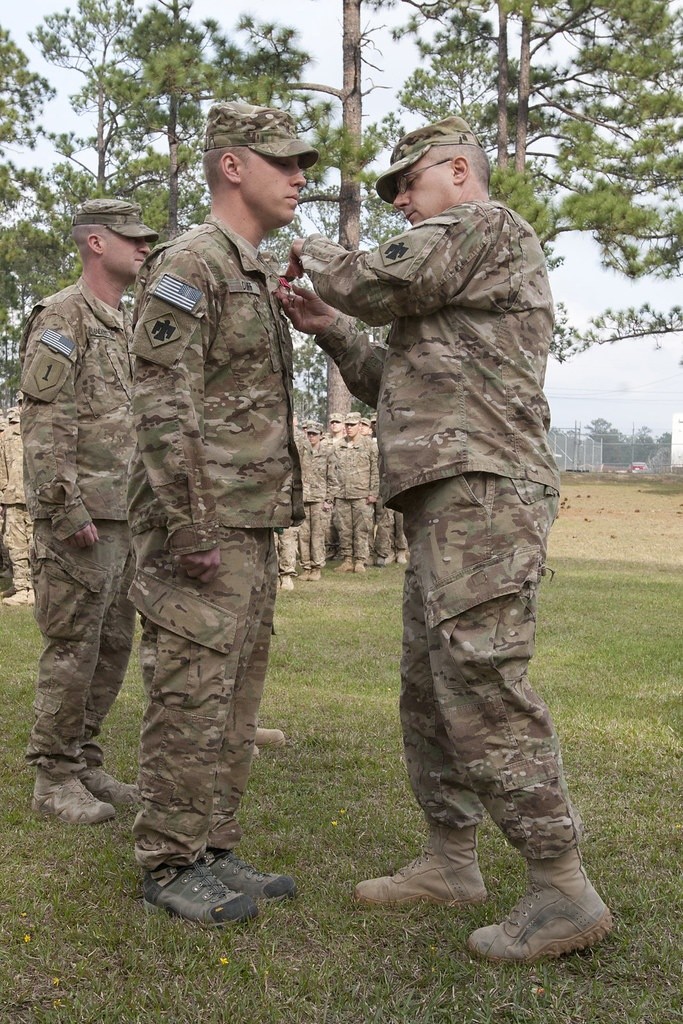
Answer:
370 414 378 422
344 413 360 425
204 99 321 169
360 418 371 427
0 407 20 431
307 423 324 434
16 390 24 402
301 420 314 428
73 199 161 243
377 115 483 205
327 413 345 423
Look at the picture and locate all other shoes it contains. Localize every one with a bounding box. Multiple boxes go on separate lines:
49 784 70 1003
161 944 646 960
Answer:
0 588 16 597
384 549 407 565
365 556 386 569
333 559 366 573
2 589 37 606
297 568 322 581
275 575 293 590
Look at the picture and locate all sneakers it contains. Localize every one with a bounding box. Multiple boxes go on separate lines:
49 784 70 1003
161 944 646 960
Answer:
142 852 300 928
468 847 613 963
354 821 488 907
31 763 140 824
253 727 285 758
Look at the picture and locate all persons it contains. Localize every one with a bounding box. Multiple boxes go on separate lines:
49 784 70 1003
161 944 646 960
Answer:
0 392 411 607
17 102 616 966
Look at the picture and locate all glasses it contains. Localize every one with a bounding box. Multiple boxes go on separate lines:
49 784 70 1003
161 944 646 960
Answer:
394 157 458 193
